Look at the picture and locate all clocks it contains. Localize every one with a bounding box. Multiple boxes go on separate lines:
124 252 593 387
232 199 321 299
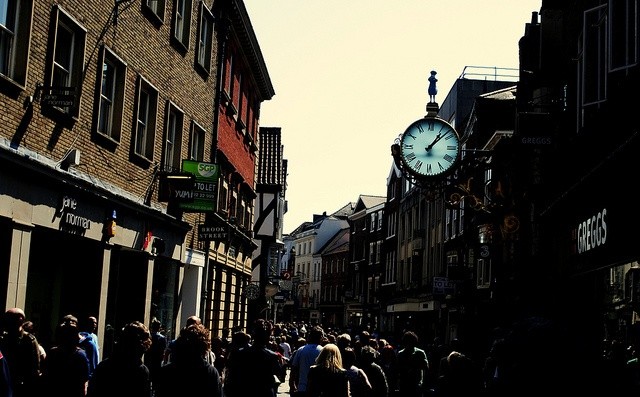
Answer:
401 117 459 180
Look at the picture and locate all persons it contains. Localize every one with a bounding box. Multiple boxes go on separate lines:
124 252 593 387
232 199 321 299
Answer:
337 326 393 396
223 318 285 396
50 314 96 395
144 313 167 382
307 342 349 395
287 325 325 396
0 306 41 396
272 317 305 355
397 329 429 396
306 319 342 343
162 324 223 396
21 312 47 364
86 321 156 396
83 313 104 364
42 318 89 396
430 326 514 397
161 314 207 363
